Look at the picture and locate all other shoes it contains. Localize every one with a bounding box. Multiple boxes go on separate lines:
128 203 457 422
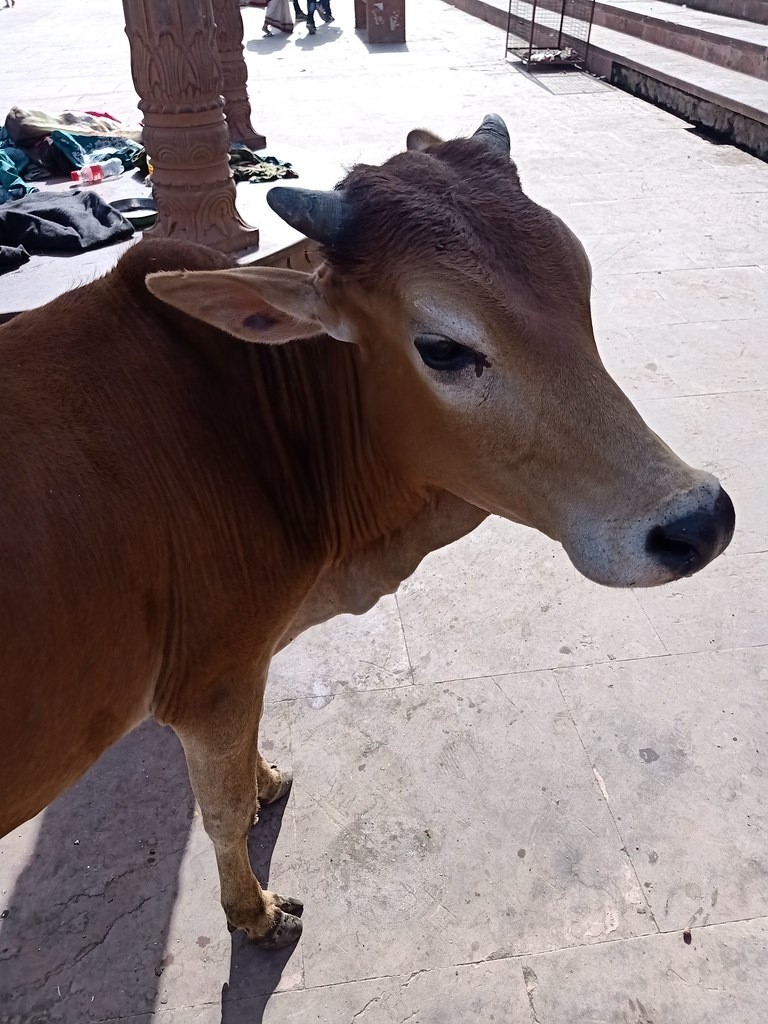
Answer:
306 23 316 34
315 1 334 21
295 11 308 21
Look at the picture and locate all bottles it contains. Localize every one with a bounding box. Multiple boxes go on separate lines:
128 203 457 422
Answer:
70 157 125 181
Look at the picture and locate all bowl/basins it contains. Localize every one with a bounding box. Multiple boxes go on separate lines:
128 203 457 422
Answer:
108 197 158 227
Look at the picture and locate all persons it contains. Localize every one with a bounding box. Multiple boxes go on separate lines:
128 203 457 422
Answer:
3 0 15 8
262 0 334 38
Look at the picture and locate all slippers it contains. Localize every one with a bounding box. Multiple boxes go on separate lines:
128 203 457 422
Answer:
263 32 274 37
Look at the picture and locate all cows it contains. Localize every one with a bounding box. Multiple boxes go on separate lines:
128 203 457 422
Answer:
0 109 736 957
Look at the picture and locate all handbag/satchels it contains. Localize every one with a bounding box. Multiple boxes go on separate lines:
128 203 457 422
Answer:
35 133 145 183
